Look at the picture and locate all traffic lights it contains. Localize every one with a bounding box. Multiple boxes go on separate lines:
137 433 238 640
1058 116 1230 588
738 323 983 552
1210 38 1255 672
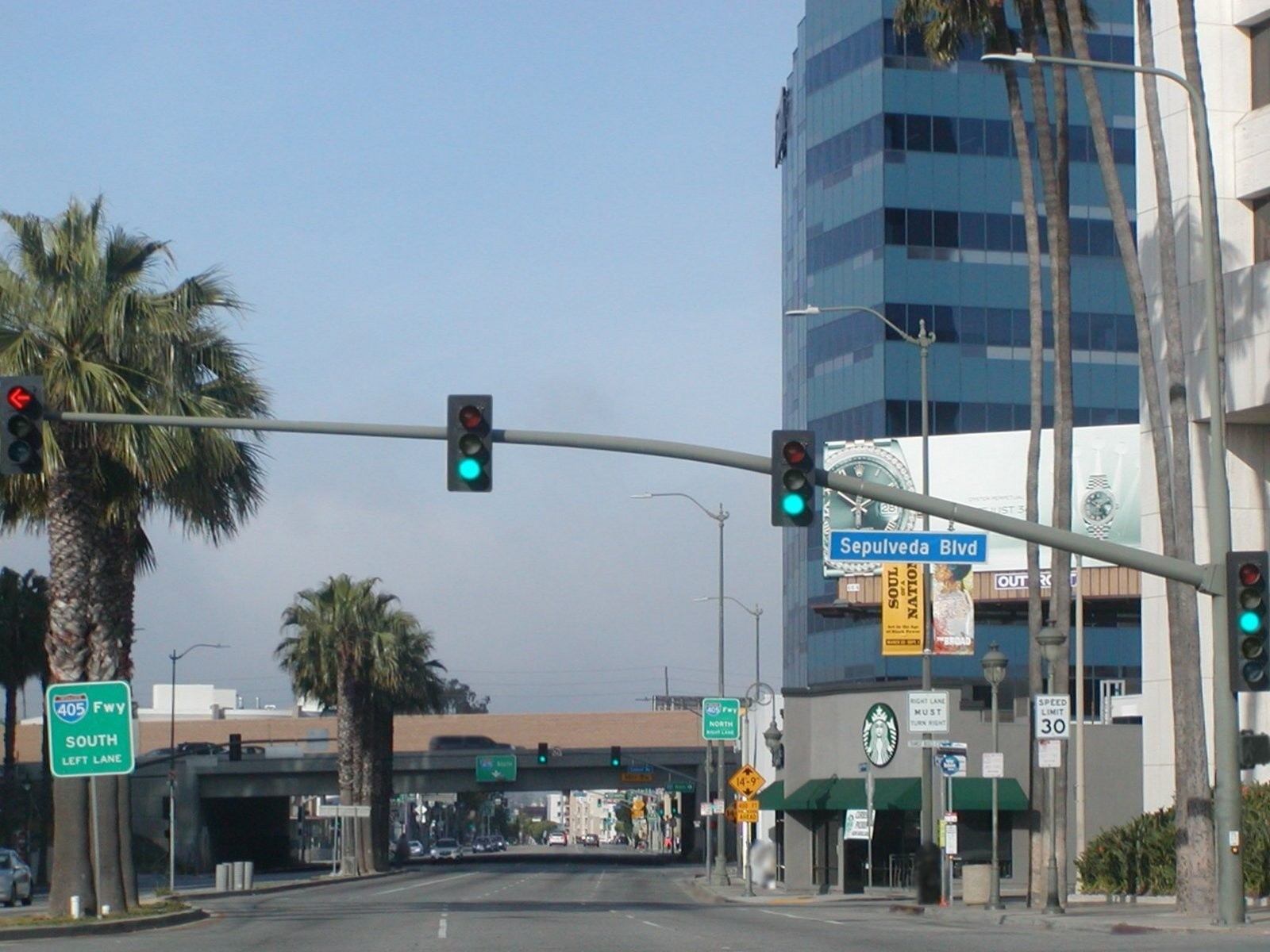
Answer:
658 800 664 816
0 378 44 472
597 799 601 807
1225 549 1270 691
671 799 680 816
610 746 622 769
770 428 818 527
446 395 495 492
538 742 548 764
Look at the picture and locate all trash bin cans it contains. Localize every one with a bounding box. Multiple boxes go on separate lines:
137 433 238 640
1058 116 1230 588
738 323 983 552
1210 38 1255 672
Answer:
962 863 994 908
214 861 254 892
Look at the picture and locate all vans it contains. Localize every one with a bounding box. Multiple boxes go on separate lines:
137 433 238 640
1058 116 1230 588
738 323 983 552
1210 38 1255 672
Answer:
428 735 515 752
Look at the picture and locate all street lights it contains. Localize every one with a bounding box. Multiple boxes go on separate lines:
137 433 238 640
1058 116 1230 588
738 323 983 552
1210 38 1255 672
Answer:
980 639 1011 912
168 642 232 892
1035 615 1069 916
630 492 733 886
636 696 713 880
692 596 763 702
978 42 1251 926
782 303 942 908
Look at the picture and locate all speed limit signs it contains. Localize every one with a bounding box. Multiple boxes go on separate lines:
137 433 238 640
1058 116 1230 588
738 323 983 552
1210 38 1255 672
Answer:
1034 694 1072 741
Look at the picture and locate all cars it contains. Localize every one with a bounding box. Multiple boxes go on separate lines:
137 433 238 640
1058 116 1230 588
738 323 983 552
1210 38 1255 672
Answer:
576 833 600 847
406 838 464 861
549 833 566 846
0 847 37 908
616 836 629 845
473 834 508 853
